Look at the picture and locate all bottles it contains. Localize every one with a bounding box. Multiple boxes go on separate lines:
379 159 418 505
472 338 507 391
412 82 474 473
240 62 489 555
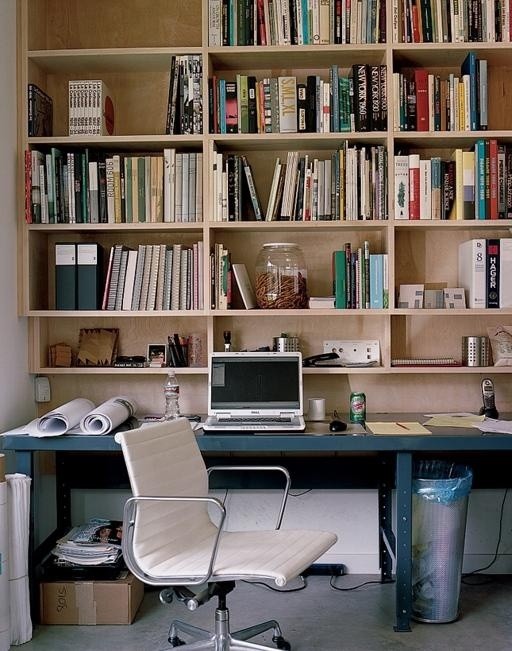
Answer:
163 369 181 419
187 332 203 367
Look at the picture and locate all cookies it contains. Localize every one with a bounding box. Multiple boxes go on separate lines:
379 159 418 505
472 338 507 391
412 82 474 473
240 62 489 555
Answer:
255 271 308 309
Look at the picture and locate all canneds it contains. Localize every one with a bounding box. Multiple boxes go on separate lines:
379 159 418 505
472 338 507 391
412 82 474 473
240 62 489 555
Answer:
349 392 367 422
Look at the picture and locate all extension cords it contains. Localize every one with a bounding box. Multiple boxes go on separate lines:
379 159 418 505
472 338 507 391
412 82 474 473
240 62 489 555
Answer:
298 564 345 577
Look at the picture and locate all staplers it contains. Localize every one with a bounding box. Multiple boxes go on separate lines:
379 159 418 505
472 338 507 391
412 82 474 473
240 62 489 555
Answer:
302 352 343 367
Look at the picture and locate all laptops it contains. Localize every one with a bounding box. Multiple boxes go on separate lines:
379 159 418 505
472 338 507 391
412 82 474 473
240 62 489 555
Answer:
203 351 306 432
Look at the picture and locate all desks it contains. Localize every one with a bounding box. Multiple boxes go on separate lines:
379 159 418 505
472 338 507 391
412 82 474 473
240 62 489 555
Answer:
1 413 512 634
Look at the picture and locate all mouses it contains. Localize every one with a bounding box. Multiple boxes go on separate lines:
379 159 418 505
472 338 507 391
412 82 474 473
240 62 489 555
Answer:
330 420 347 432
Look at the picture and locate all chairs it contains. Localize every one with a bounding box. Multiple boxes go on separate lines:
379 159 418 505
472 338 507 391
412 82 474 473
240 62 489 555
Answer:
112 416 338 651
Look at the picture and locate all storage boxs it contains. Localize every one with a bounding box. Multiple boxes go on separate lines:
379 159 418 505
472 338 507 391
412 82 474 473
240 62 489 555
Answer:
38 571 145 626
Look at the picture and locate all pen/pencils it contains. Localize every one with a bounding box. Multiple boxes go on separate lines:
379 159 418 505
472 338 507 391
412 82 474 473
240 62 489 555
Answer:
168 333 188 367
395 422 411 431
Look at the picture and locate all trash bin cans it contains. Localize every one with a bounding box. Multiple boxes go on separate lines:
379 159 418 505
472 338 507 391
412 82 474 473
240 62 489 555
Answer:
412 458 473 624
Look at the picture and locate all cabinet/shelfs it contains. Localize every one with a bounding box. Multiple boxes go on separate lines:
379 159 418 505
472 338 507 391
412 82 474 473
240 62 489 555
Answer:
15 0 512 376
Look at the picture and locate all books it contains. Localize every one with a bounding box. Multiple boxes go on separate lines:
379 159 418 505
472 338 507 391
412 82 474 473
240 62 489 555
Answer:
27 55 203 137
50 517 124 566
209 65 389 221
391 357 455 366
309 241 389 310
458 238 512 310
209 243 257 310
208 1 512 46
392 51 512 220
24 148 204 310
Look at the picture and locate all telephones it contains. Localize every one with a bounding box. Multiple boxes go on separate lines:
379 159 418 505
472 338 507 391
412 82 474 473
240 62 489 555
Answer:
479 377 499 419
